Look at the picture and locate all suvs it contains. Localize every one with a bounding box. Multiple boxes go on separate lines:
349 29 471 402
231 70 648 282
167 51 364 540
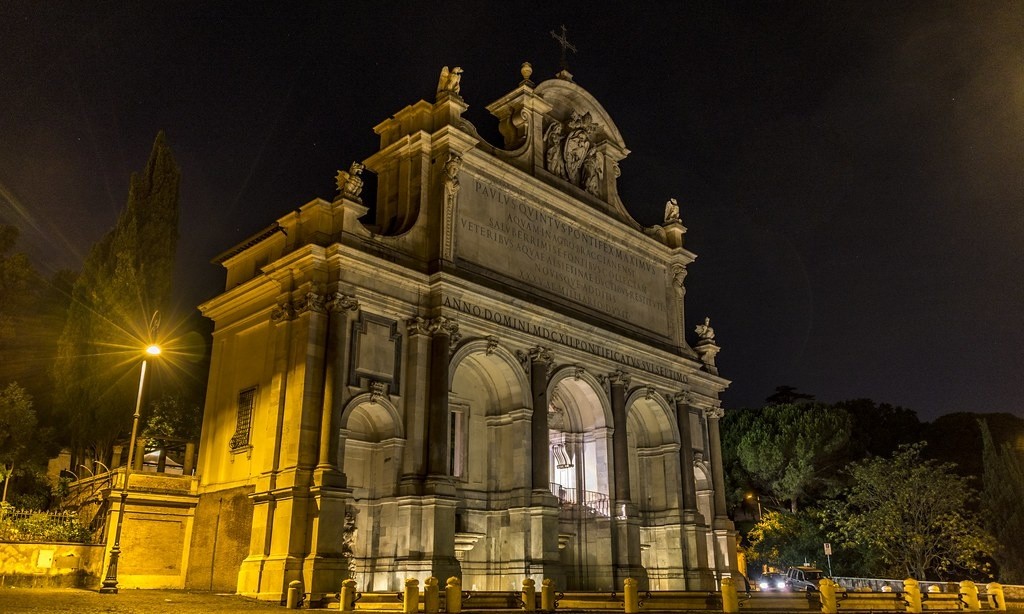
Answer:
785 566 825 592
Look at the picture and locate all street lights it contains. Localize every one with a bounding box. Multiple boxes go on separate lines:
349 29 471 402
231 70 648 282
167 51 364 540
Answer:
747 493 766 526
100 340 165 594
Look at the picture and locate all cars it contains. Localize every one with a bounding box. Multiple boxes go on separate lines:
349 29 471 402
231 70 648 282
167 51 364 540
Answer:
759 572 785 592
